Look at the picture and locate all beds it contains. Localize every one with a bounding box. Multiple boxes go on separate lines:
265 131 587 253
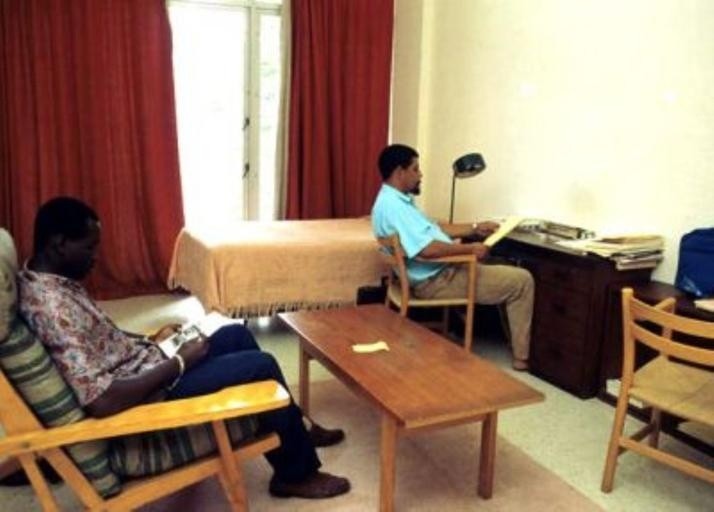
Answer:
183 216 411 327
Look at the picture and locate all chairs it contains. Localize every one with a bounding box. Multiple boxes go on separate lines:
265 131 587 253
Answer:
374 234 478 352
599 286 713 512
0 223 291 510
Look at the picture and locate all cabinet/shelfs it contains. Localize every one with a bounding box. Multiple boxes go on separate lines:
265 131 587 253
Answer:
446 234 663 402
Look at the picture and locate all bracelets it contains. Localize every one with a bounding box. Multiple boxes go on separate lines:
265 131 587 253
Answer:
169 353 184 376
471 220 478 238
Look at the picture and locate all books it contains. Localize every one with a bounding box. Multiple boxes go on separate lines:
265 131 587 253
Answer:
158 310 248 363
554 231 664 272
483 215 523 248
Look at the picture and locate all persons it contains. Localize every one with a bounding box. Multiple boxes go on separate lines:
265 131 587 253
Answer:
367 145 534 373
13 195 352 499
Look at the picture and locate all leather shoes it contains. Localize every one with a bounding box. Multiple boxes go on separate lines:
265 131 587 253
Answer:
307 423 344 445
269 470 349 498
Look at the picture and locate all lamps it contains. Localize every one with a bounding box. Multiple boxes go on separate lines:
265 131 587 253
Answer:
443 152 488 224
